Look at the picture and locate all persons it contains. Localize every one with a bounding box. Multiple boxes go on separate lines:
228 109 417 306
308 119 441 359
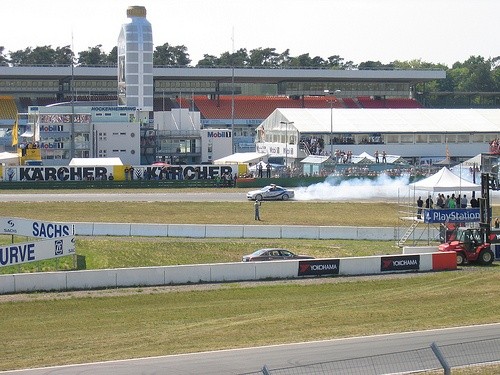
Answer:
416 196 423 219
436 193 487 208
254 200 262 221
18 111 472 189
494 218 499 228
425 195 433 208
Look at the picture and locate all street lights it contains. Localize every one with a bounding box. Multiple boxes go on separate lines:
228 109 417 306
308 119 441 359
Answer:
324 89 341 160
280 121 295 169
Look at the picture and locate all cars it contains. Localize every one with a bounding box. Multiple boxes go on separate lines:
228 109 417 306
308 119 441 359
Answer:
241 248 316 262
246 184 295 201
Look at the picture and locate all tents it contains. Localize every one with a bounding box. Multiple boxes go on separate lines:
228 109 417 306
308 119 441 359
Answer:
408 167 482 207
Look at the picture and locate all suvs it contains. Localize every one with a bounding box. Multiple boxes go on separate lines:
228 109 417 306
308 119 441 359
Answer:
436 226 495 267
